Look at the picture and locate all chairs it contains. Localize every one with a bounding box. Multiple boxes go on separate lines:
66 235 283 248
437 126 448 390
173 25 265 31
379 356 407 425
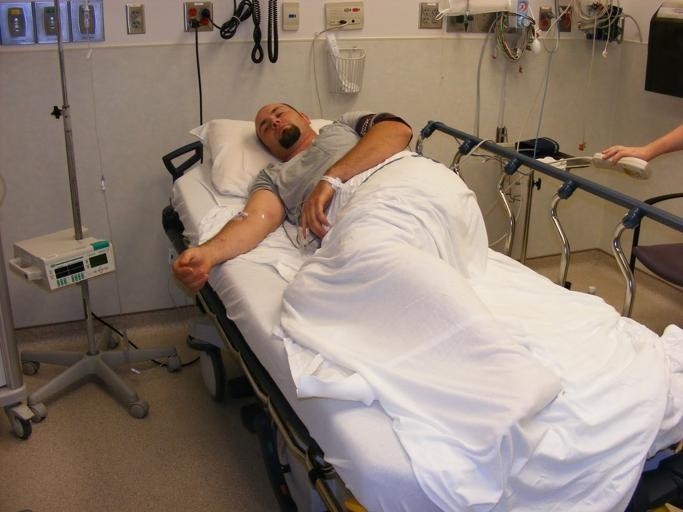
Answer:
629 194 682 283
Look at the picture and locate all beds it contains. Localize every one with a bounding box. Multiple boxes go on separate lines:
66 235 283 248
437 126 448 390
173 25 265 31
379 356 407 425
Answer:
162 120 682 512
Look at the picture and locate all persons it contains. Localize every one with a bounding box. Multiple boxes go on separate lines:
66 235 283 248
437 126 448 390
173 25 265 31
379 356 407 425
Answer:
602 124 683 169
171 102 413 295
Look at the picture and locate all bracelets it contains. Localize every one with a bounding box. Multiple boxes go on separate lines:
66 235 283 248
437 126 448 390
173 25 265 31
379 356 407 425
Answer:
319 175 343 189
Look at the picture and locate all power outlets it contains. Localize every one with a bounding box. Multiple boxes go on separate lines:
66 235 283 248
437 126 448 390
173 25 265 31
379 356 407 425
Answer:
420 2 442 28
539 5 552 32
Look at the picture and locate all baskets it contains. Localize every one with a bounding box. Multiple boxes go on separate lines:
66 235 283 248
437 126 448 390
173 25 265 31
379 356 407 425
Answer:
329 49 366 94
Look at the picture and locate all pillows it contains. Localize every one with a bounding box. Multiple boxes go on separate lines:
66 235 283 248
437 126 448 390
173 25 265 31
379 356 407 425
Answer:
189 116 333 198
